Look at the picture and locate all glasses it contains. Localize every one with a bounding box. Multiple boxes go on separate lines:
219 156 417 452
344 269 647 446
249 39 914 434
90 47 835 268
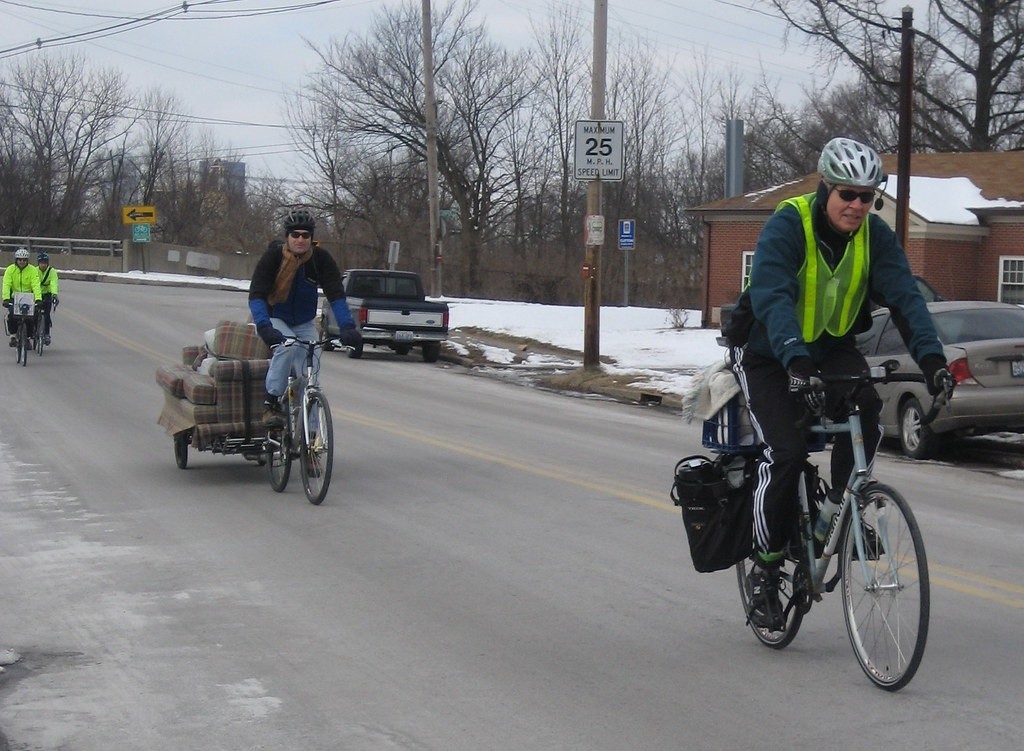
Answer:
289 230 312 239
830 183 875 204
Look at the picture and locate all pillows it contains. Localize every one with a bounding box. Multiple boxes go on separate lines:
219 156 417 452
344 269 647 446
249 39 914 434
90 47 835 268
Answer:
191 320 272 375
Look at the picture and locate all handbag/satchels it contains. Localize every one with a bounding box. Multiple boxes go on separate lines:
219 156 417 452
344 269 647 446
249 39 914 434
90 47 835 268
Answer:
671 456 756 573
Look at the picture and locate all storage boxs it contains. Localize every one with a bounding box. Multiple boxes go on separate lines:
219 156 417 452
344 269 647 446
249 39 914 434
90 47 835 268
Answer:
698 389 827 455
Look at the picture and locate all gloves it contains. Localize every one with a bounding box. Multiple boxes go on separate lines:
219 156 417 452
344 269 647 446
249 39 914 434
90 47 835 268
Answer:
921 354 957 406
787 356 826 416
261 326 286 346
341 324 363 352
36 301 45 308
51 296 60 305
3 299 12 308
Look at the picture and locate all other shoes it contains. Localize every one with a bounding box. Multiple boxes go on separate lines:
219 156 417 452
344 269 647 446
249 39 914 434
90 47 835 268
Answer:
9 337 16 347
45 337 50 345
27 340 32 349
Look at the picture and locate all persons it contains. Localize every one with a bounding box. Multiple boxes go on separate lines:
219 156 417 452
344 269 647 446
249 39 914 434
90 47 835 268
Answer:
2 248 42 350
729 136 955 630
248 210 362 477
34 254 59 345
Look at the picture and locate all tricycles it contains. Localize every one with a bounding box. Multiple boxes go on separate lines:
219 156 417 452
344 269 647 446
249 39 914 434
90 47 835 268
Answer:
172 329 355 505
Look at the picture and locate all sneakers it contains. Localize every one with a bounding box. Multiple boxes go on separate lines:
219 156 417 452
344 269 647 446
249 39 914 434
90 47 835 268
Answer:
852 525 881 556
262 404 285 427
309 454 322 477
744 563 785 625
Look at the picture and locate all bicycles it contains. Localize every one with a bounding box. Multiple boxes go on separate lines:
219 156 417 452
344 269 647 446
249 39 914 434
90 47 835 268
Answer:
712 356 956 692
35 294 57 357
3 302 38 366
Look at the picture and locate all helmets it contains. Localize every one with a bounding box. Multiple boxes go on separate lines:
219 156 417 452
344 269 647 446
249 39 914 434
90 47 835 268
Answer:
37 254 49 260
15 249 30 258
817 137 884 185
284 210 315 229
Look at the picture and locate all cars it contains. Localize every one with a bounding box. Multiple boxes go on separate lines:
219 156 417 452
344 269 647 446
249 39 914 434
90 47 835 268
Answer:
852 299 1024 459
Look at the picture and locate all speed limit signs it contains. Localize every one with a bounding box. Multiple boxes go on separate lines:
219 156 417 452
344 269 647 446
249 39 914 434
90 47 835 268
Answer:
574 120 624 182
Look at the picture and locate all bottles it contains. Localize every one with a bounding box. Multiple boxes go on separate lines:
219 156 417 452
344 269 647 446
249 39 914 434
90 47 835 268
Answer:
814 489 844 542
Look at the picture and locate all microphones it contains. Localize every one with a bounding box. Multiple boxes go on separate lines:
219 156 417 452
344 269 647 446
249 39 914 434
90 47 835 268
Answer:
874 180 888 210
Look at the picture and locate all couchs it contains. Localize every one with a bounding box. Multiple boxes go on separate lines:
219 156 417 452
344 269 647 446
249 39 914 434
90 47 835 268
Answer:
157 312 323 450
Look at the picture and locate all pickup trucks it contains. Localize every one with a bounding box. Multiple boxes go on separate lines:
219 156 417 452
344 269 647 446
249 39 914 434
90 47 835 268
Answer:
317 268 450 363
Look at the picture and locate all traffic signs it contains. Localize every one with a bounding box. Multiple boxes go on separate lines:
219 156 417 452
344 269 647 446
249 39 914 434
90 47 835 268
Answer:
121 206 156 224
